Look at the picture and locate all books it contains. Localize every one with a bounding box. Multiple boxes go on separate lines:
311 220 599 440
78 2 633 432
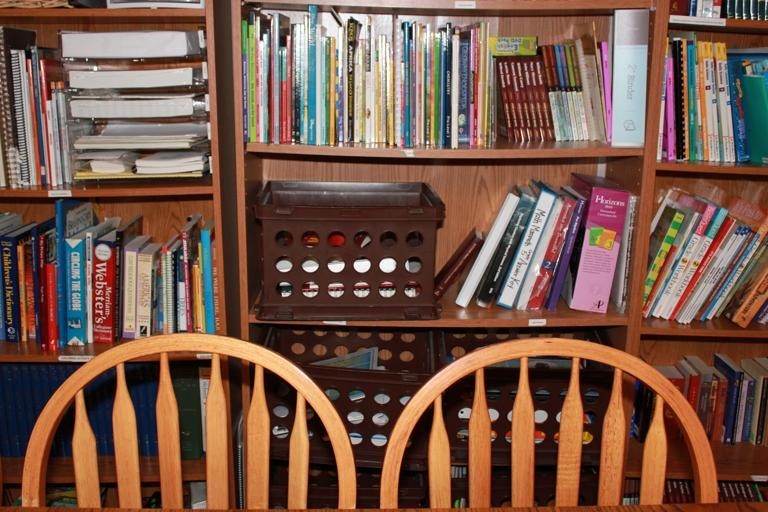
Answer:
0 21 211 190
434 170 639 315
450 466 470 509
620 477 766 503
10 481 208 509
632 352 768 445
240 5 650 151
641 187 768 328
657 0 768 166
3 201 218 349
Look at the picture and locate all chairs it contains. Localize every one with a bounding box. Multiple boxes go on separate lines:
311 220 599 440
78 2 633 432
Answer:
21 332 358 509
379 337 719 509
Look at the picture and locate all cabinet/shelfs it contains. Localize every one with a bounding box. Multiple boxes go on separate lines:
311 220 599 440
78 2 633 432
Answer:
232 1 667 512
620 0 768 505
1 1 242 512
